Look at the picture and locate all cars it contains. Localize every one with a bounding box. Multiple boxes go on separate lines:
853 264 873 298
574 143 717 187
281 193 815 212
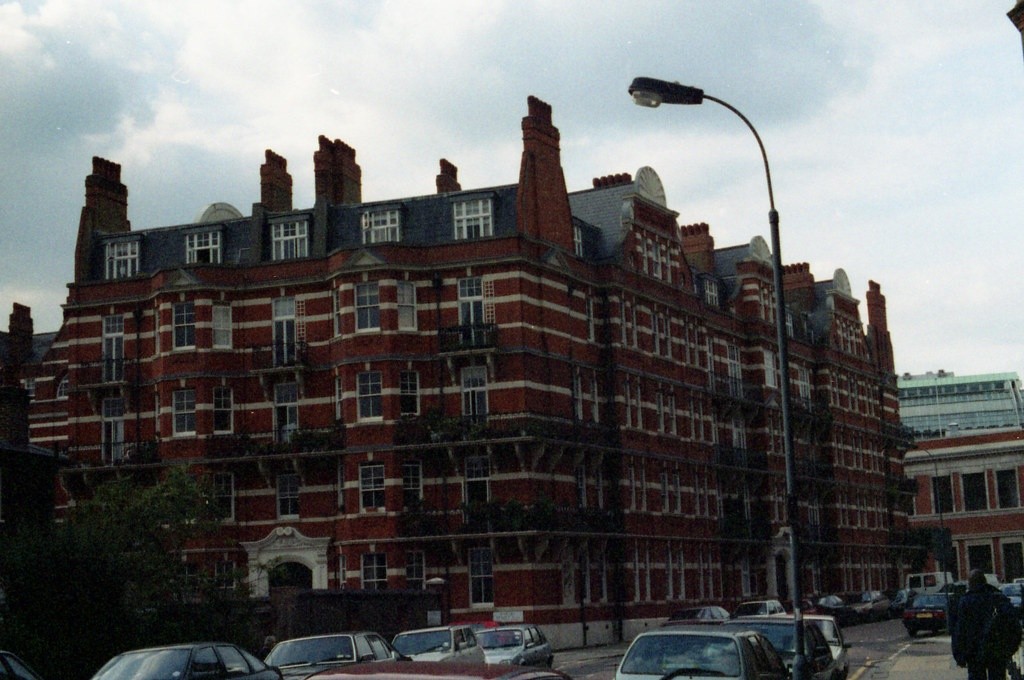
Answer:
91 642 284 680
732 599 788 619
612 626 793 680
718 613 853 680
389 624 486 663
300 658 575 680
797 580 1024 638
473 624 555 670
262 629 414 680
661 605 733 629
0 651 43 680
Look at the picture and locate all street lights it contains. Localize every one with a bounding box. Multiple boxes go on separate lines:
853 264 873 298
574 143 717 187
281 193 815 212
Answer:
897 443 947 572
627 75 810 680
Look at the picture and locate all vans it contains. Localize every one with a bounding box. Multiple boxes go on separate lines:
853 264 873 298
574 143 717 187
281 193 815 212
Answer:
905 572 953 593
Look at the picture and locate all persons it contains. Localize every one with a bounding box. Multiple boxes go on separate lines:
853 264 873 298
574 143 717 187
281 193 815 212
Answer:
951 568 1009 680
257 635 276 662
